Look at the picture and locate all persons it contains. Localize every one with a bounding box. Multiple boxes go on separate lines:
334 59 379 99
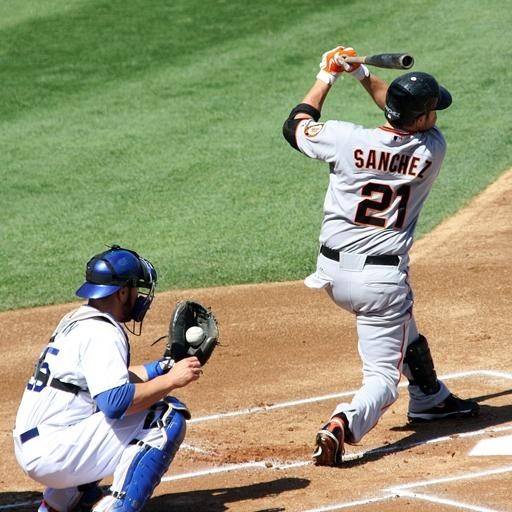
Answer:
282 44 480 466
12 243 203 511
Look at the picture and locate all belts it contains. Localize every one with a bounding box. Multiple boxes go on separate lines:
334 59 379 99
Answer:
319 245 400 265
19 426 40 445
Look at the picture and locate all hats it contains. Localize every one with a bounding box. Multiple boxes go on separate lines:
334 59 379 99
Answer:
384 71 453 126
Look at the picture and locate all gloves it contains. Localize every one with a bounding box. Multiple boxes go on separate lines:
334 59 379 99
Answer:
316 46 344 86
334 46 370 82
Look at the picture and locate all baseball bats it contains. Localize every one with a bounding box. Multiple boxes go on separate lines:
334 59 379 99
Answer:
344 52 415 69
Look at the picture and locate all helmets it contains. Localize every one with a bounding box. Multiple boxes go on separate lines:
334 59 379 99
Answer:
76 245 158 336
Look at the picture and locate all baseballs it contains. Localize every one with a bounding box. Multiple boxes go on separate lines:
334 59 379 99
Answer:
185 326 205 346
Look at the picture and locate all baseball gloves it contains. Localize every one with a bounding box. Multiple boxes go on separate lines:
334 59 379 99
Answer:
163 300 220 367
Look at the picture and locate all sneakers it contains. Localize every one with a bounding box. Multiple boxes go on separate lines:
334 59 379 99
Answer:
312 416 348 467
406 392 480 422
